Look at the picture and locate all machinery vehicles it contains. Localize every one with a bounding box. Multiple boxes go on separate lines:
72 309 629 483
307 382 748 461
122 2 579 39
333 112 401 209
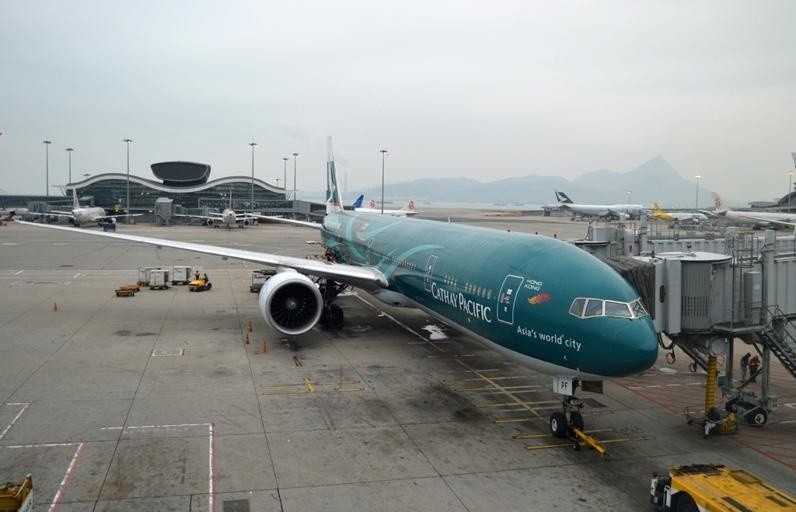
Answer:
650 462 796 512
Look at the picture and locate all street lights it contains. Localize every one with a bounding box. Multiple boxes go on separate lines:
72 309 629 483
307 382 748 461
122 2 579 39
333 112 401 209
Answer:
43 141 51 201
65 148 73 184
292 153 298 200
626 191 632 204
282 158 289 190
696 175 701 213
248 142 258 226
82 173 90 179
275 178 280 187
788 170 793 213
123 138 133 225
380 149 387 215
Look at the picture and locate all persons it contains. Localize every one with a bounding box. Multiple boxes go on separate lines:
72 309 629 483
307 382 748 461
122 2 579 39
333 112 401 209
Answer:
749 356 762 382
739 352 751 382
194 270 200 280
202 273 209 284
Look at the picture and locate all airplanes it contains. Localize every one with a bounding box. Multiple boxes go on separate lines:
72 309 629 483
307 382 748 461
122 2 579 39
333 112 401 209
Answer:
11 131 659 438
27 188 144 228
710 192 796 231
172 190 283 229
647 202 719 226
541 188 645 222
354 200 418 218
286 194 364 211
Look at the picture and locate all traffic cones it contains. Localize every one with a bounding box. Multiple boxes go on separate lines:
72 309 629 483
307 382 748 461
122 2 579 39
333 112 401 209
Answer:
246 320 253 333
53 302 58 312
243 333 250 345
259 341 267 353
